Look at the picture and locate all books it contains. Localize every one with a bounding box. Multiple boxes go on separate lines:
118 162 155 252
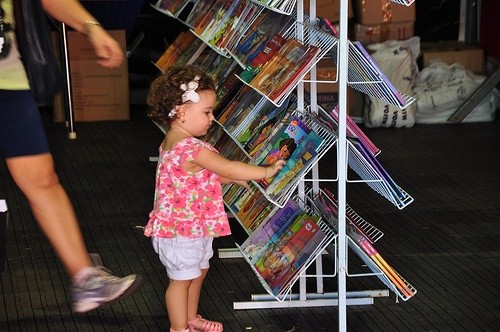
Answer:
151 0 413 298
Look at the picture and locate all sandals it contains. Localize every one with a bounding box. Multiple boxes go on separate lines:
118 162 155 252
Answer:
170 315 223 332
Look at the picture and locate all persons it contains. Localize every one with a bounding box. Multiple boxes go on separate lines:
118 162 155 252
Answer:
143 64 286 332
0 1 142 314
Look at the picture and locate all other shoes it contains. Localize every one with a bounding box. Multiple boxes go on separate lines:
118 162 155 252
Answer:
69 253 143 314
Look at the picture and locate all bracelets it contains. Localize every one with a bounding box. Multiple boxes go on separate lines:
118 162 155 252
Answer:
79 19 101 26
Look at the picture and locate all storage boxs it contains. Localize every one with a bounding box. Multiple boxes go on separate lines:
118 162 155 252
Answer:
304 0 484 121
67 28 132 122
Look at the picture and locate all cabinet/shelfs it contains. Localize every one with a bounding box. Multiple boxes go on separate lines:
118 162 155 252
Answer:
148 0 417 332
